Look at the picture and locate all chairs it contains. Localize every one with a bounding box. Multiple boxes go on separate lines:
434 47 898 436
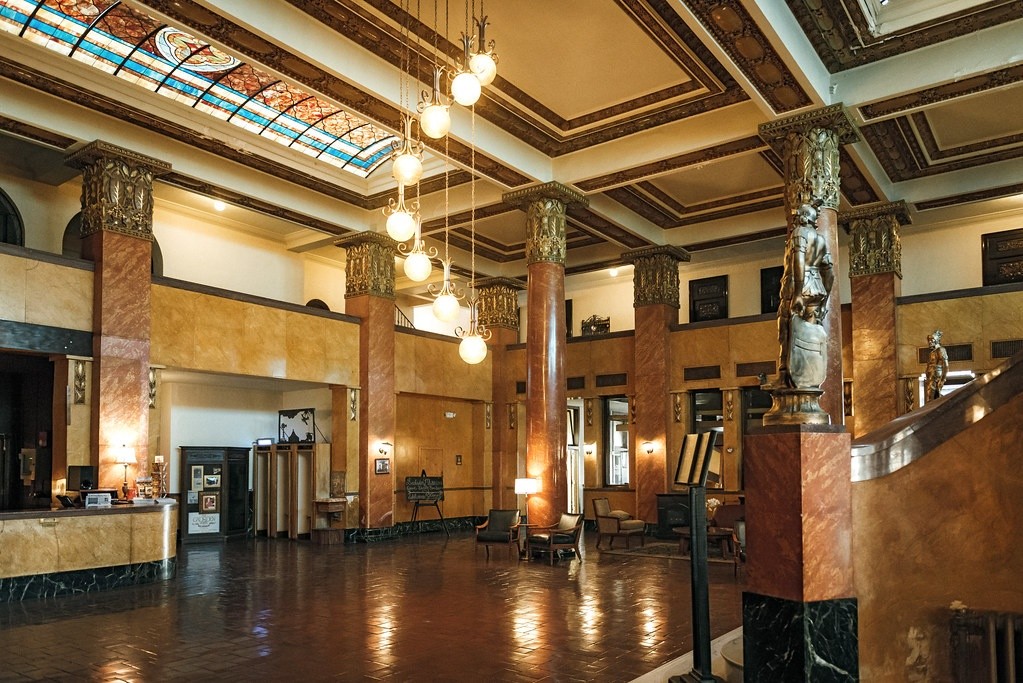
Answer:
592 496 645 550
475 508 521 561
732 522 746 575
527 511 584 566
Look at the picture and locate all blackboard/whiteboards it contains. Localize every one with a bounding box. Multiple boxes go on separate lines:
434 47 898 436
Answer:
405 476 445 501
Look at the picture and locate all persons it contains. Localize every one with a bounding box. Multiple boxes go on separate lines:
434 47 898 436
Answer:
768 197 835 389
706 498 741 545
924 330 949 405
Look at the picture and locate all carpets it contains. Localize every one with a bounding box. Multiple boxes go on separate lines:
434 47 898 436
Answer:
601 543 736 564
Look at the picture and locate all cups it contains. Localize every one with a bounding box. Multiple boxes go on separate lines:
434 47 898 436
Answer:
126 488 135 501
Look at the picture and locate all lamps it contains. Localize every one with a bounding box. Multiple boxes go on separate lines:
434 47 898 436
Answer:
585 441 592 455
514 477 537 561
382 0 499 366
115 444 138 501
379 441 393 456
642 440 655 455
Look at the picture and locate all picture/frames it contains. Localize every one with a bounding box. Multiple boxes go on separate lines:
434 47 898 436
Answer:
204 474 221 487
199 492 220 513
375 458 391 475
191 465 204 492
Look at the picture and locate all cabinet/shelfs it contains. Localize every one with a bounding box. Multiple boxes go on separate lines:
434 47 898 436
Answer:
151 455 167 500
179 444 249 543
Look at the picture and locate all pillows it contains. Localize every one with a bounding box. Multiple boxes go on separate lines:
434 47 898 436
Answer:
608 510 629 521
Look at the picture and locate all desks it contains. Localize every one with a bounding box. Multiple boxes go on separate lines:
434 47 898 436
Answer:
670 527 733 558
518 523 538 561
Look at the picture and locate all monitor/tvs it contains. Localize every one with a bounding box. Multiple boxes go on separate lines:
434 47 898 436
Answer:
68 465 94 491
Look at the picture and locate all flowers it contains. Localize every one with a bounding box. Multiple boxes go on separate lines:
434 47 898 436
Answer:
705 497 720 519
